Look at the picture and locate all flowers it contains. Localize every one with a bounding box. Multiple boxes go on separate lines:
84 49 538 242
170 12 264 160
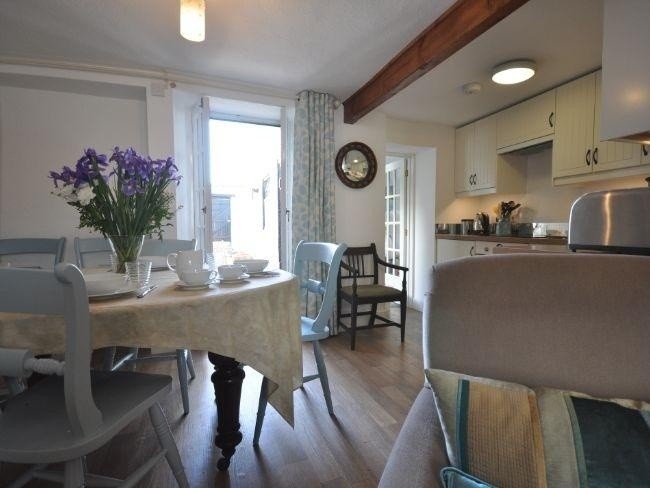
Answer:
47 144 184 274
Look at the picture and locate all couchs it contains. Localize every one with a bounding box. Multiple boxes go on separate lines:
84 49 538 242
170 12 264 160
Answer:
377 252 650 487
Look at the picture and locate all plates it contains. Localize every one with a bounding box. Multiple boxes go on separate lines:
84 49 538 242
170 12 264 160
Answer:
175 281 215 291
89 283 132 302
216 275 250 284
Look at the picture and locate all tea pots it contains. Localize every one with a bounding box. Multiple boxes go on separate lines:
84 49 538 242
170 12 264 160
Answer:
168 249 205 280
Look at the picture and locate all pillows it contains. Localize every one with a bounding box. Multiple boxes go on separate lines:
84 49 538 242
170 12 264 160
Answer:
425 365 650 487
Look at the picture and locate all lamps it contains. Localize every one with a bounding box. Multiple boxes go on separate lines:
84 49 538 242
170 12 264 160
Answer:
178 0 206 42
489 58 538 87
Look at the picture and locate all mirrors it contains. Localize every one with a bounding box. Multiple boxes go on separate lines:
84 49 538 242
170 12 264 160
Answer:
336 141 377 188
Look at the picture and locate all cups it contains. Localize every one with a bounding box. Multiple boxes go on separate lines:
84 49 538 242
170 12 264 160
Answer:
462 219 473 234
218 265 248 278
106 251 118 273
207 253 226 268
124 260 153 288
181 269 217 285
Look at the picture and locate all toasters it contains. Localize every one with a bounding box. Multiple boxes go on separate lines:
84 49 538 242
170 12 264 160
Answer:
566 187 650 256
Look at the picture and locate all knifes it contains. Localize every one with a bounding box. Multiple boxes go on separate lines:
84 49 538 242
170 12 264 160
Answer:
138 285 159 298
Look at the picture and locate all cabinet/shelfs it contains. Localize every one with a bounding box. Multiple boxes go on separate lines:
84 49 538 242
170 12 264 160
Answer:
495 88 554 153
553 66 643 186
435 236 572 264
453 118 526 197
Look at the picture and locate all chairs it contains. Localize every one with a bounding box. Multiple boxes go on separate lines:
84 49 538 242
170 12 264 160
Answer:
0 236 66 271
236 238 348 446
0 262 192 488
336 241 409 350
75 235 193 413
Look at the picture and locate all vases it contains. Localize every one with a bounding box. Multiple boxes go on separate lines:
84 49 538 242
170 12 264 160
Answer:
104 231 146 274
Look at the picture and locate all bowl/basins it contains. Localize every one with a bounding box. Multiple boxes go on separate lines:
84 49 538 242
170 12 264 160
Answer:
85 273 128 294
235 260 269 273
436 223 461 233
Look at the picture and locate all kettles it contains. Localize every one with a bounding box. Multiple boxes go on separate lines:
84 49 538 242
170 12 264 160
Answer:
473 212 490 235
